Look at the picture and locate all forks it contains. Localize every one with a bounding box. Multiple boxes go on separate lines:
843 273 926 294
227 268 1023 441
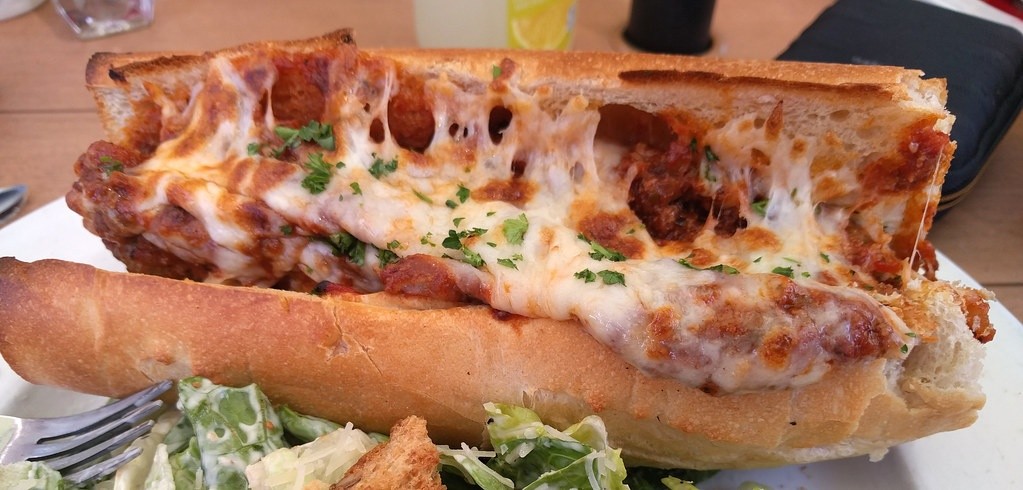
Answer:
0 378 172 486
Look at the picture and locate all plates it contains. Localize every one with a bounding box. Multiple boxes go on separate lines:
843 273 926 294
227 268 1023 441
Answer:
0 194 1022 490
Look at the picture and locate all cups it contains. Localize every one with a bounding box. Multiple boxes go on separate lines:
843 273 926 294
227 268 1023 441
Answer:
412 0 579 56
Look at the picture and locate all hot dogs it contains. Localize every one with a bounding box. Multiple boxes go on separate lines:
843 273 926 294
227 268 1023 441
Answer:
0 26 997 489
